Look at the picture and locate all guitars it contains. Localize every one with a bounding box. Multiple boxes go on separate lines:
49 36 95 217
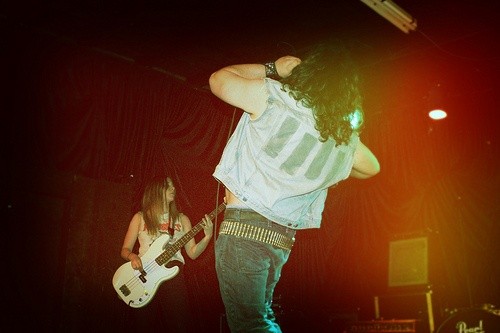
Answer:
111 196 228 309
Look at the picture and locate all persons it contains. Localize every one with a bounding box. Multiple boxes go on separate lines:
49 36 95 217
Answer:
121 176 213 333
209 41 380 333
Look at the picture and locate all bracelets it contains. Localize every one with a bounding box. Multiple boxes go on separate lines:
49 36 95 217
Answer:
127 252 134 261
265 61 284 81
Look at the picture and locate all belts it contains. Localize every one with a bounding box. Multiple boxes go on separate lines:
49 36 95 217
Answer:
219 222 296 252
